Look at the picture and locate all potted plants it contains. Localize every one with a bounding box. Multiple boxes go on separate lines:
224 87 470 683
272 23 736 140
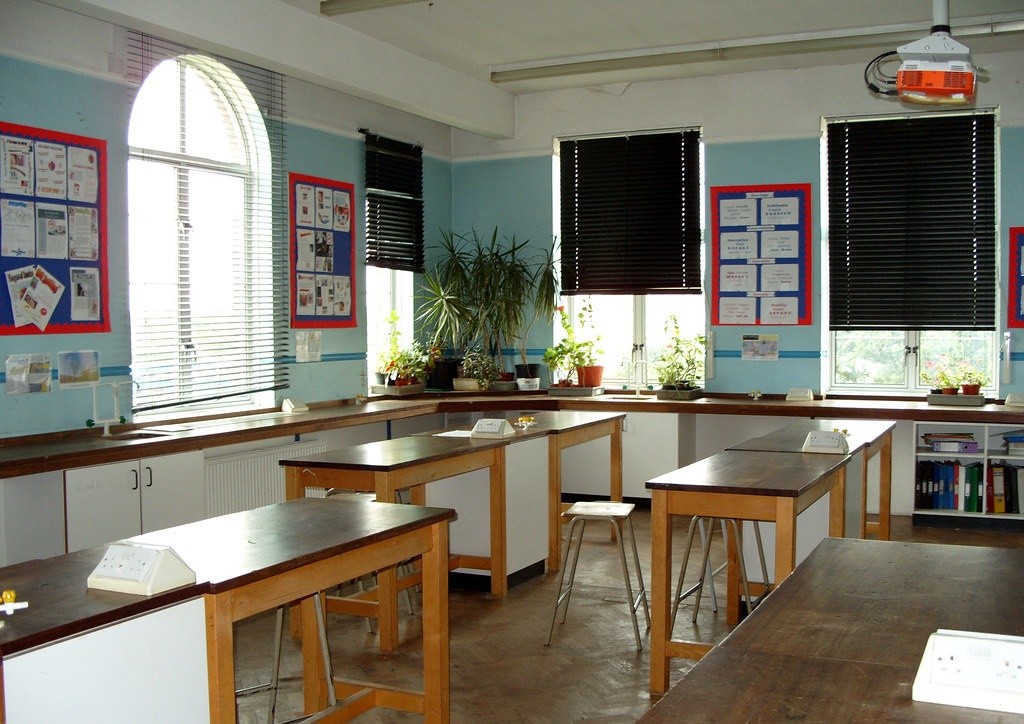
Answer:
574 297 604 386
407 223 564 391
651 316 710 391
962 367 989 395
940 372 961 395
921 373 945 394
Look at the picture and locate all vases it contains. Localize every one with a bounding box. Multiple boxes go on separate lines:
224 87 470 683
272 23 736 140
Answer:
559 379 573 387
376 372 387 384
408 377 418 385
396 378 408 387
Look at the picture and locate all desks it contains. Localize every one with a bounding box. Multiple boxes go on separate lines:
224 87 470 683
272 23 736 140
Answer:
645 450 852 696
426 409 627 574
737 421 897 606
278 434 505 654
718 536 1024 668
636 651 1024 724
0 496 457 724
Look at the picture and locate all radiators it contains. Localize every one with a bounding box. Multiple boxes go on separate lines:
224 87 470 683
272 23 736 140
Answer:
204 439 329 519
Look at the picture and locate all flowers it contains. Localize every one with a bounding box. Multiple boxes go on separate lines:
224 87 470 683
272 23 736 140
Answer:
544 303 576 379
380 310 444 379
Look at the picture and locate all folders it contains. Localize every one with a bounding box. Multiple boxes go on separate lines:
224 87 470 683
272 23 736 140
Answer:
988 465 1024 514
932 442 978 453
915 460 983 513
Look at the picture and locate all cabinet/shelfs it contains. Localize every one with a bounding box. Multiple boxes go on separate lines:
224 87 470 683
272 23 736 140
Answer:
911 421 1024 522
0 450 205 568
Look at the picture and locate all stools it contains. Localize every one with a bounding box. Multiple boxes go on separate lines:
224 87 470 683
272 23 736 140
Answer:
236 592 338 724
668 515 771 639
330 490 415 630
544 501 650 650
